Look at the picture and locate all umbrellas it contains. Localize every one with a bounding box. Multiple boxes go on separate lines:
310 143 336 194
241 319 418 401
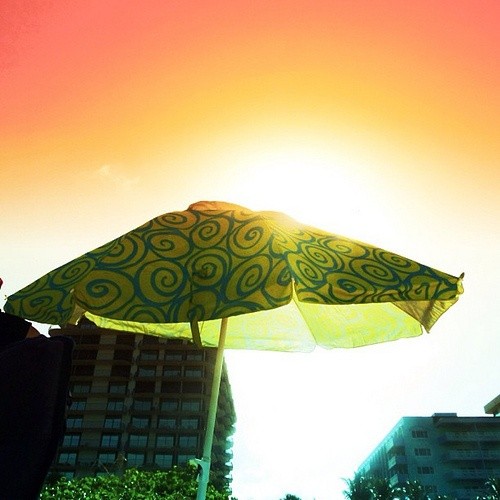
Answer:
2 198 467 500
